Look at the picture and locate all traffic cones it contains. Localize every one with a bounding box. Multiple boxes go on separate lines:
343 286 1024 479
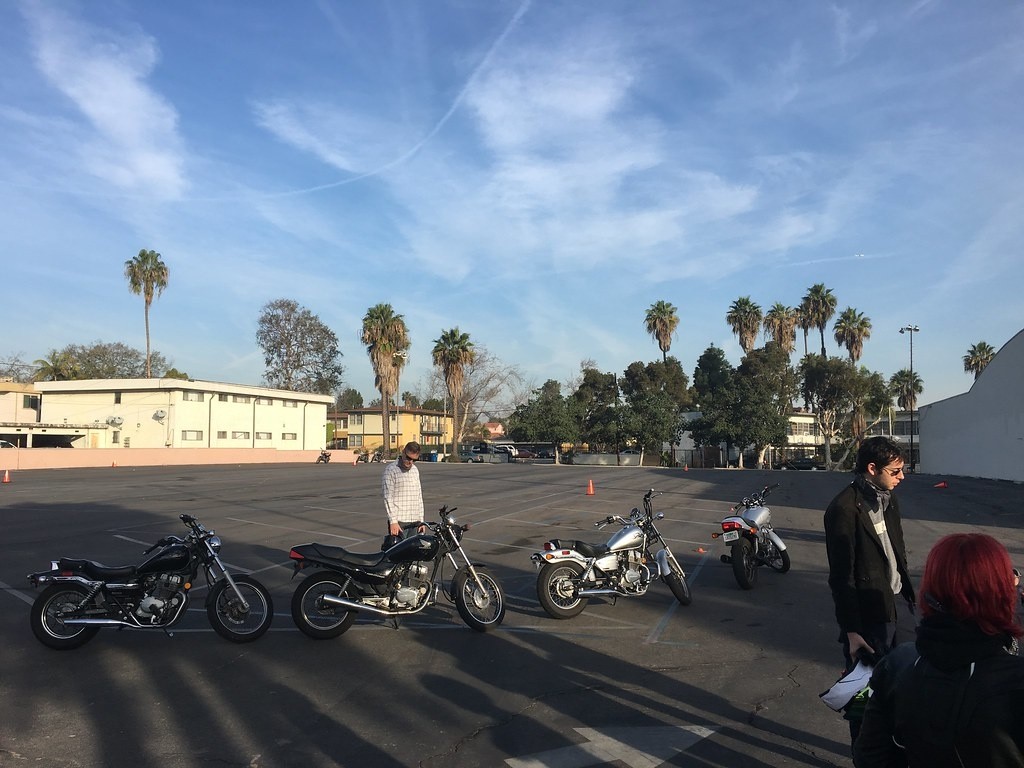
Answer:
111 460 116 468
1 469 12 483
933 481 947 489
585 478 595 495
684 463 689 472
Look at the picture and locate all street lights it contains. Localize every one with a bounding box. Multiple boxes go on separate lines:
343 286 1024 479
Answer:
899 324 921 475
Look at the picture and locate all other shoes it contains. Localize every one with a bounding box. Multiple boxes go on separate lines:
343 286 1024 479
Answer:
889 732 908 751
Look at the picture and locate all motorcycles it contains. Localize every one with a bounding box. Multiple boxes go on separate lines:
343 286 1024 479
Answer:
27 513 274 644
315 447 332 464
713 483 791 586
352 449 389 464
277 503 506 642
528 486 695 622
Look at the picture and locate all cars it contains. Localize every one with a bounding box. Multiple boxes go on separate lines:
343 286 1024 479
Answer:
415 438 643 463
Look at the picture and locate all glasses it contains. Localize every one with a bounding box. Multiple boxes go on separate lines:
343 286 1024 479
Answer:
1011 567 1022 581
404 452 420 462
882 466 903 477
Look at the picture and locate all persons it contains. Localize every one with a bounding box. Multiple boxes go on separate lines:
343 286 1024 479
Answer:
852 532 1024 768
824 436 921 762
382 441 429 574
